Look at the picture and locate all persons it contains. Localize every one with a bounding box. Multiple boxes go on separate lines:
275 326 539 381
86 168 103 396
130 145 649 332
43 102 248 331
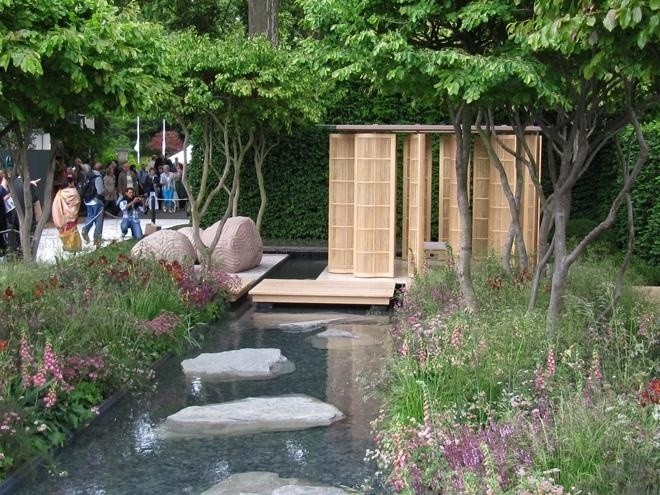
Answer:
1 153 183 262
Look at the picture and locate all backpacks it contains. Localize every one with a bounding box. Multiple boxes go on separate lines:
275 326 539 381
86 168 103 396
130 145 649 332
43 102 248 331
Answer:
80 170 102 203
